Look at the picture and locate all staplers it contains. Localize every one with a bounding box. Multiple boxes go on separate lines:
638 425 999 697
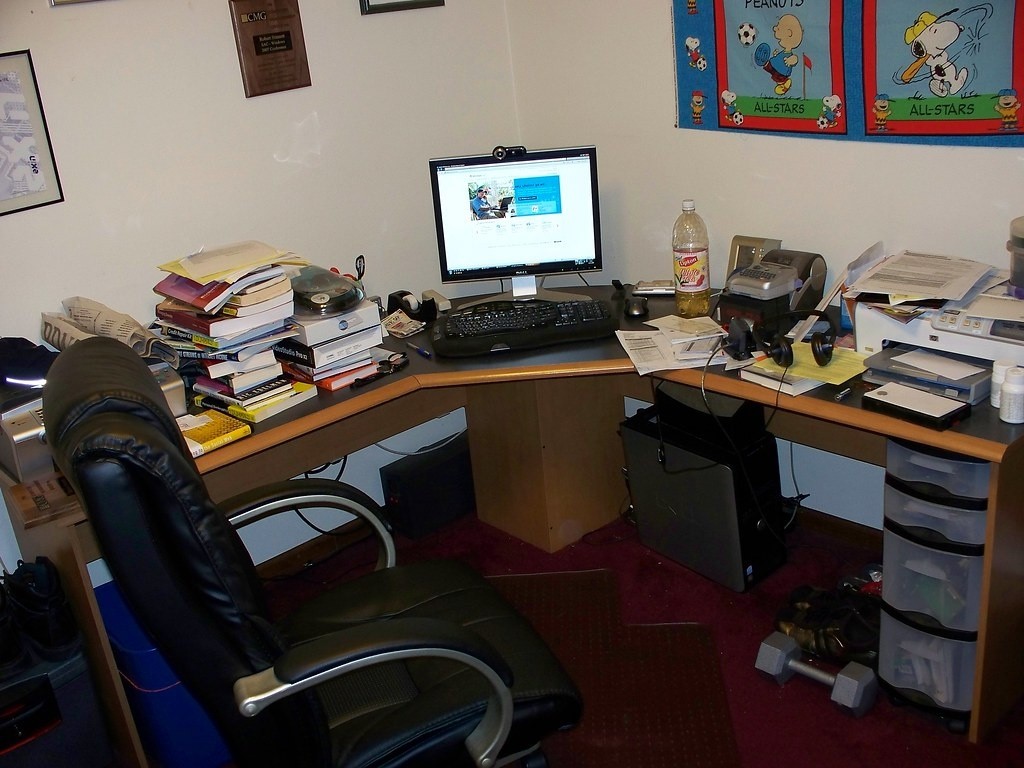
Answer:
421 289 452 316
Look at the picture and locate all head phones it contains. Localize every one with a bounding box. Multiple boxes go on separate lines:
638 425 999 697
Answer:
754 308 837 367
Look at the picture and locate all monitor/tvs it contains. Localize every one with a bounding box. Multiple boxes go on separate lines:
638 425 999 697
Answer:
429 144 603 311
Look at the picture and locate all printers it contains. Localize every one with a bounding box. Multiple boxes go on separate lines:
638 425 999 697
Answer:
854 277 1024 406
0 353 189 484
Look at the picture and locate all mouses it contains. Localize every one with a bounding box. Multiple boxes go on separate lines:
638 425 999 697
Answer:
624 297 649 318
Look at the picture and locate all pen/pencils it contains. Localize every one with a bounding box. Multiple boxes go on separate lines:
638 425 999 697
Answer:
407 342 431 358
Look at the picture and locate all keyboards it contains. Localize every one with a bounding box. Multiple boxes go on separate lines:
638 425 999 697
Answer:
430 297 621 357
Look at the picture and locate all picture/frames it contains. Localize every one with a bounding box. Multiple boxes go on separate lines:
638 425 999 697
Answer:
359 0 445 15
0 49 66 217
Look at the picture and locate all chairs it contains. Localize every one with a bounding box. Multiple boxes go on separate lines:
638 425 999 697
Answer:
44 337 578 768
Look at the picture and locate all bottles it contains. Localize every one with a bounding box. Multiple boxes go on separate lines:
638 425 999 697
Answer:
1005 216 1024 291
998 367 1024 423
672 199 711 315
990 358 1017 408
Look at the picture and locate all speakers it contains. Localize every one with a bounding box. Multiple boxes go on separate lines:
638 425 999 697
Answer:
379 430 479 540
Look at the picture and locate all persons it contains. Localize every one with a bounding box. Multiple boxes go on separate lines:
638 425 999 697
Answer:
473 189 499 218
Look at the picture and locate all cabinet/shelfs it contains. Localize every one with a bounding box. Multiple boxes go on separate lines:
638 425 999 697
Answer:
875 434 989 726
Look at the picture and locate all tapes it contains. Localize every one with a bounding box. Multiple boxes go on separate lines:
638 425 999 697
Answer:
403 294 418 310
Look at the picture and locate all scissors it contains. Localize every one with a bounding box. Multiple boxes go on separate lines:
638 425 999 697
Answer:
330 267 357 282
356 255 365 279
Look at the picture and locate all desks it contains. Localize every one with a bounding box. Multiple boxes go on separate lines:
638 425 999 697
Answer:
1 285 1024 745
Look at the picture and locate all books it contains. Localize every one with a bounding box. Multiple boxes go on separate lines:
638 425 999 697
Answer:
153 264 404 423
180 409 251 457
8 470 82 527
739 365 824 398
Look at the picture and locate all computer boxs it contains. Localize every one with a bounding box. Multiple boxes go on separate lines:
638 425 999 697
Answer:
618 402 789 592
